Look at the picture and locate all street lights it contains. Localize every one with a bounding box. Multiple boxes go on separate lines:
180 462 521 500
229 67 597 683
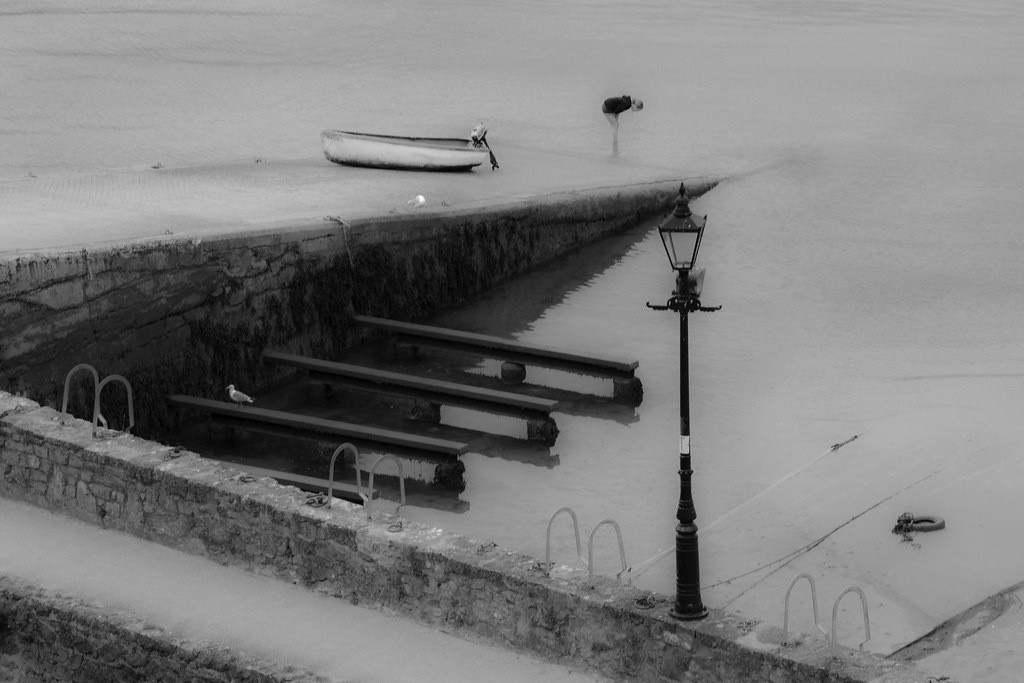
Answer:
646 183 721 621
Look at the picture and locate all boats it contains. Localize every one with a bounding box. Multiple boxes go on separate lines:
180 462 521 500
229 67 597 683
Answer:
321 121 500 171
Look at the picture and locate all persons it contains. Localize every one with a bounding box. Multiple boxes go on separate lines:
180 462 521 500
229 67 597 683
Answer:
602 95 643 142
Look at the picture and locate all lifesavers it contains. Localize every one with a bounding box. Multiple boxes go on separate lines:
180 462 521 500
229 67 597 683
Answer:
908 514 946 532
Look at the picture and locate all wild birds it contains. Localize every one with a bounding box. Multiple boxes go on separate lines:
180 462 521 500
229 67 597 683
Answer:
225 384 253 408
